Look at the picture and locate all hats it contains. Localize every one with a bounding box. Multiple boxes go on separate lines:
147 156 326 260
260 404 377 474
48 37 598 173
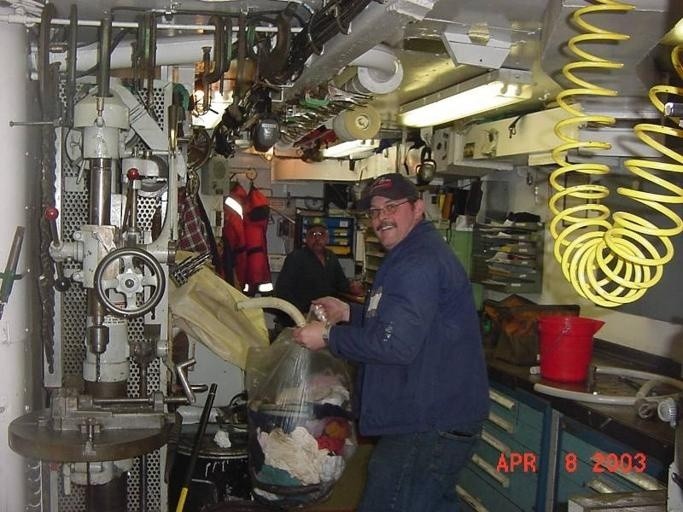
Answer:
348 173 419 211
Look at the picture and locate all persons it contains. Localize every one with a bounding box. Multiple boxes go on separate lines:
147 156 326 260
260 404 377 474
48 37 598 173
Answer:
272 226 366 331
293 172 488 512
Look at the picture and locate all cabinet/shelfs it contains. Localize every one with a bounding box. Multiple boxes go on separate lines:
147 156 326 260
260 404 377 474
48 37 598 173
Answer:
545 409 669 512
295 184 543 293
447 378 550 512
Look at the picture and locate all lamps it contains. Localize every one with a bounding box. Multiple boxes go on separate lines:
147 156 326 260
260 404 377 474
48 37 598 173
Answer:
319 135 380 159
398 67 534 128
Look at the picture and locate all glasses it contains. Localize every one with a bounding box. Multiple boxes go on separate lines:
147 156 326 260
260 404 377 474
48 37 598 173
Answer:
367 198 423 220
305 231 328 239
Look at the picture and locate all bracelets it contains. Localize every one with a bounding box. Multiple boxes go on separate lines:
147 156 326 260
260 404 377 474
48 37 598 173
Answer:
322 321 332 341
